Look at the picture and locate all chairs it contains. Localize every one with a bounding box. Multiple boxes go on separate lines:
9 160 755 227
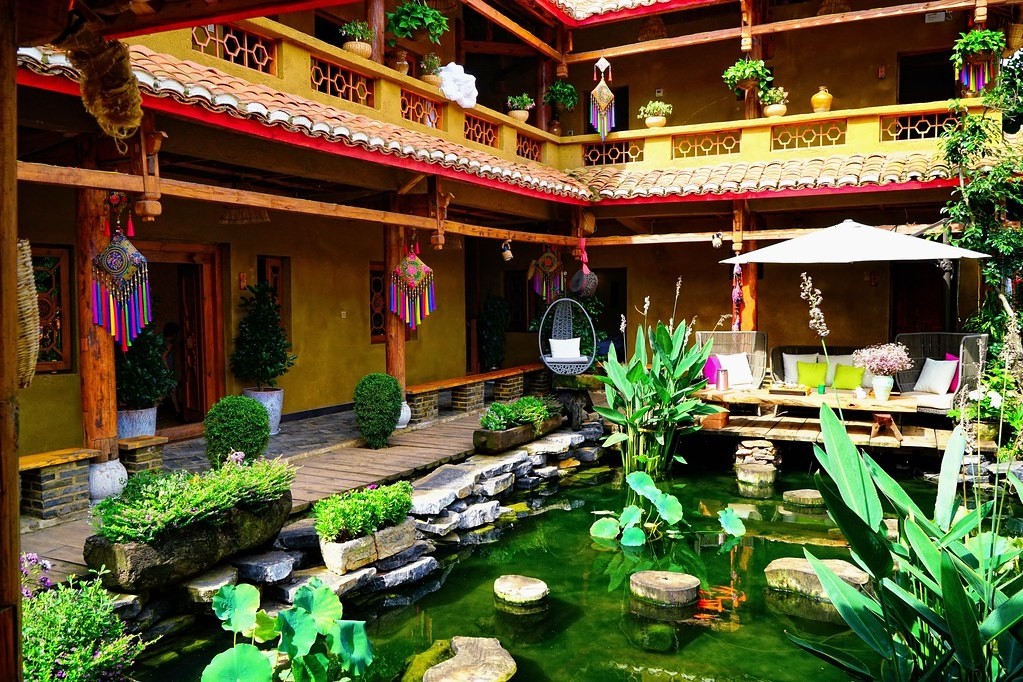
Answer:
695 330 767 417
895 331 988 426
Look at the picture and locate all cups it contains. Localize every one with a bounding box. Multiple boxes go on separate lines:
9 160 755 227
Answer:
818 384 825 395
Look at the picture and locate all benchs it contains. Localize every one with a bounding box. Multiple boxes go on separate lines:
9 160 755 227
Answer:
769 345 871 418
19 435 169 520
405 362 552 421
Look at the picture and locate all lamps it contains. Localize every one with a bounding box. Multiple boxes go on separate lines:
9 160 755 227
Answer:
502 238 513 261
711 233 723 249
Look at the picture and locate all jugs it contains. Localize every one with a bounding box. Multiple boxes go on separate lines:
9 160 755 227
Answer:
716 369 728 391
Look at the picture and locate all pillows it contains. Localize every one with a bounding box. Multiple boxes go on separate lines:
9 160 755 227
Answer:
702 353 960 395
548 337 581 358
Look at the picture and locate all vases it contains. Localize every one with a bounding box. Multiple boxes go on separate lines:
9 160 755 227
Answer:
872 375 894 402
508 110 529 122
969 420 1000 440
84 488 415 590
342 40 443 87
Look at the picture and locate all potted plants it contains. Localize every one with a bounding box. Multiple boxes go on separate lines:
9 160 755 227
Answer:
544 80 578 114
472 393 562 455
383 0 450 49
722 58 774 97
636 100 673 127
758 87 790 116
948 28 1007 70
117 277 300 439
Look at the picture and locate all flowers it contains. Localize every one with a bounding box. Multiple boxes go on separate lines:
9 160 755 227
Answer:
87 444 415 542
946 352 1023 430
853 342 914 375
339 19 444 74
507 93 535 110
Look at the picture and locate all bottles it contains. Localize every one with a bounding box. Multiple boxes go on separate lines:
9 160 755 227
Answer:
810 86 833 113
389 52 409 75
548 120 562 137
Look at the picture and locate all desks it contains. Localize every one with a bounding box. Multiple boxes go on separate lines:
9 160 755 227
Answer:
692 388 918 441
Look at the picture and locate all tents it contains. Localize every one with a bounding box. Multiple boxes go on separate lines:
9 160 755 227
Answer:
719 218 991 331
